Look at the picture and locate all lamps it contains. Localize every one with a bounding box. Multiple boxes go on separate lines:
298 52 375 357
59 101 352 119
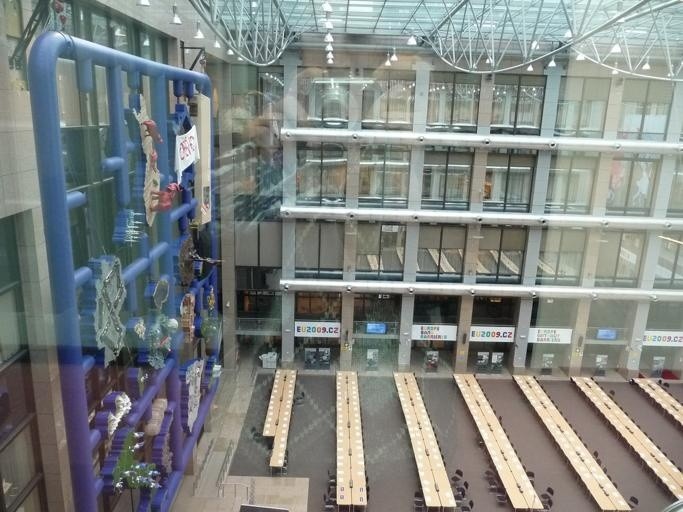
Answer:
382 18 421 66
317 0 334 66
471 15 677 77
135 1 243 64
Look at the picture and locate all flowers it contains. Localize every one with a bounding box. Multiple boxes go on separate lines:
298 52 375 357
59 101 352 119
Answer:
111 430 162 493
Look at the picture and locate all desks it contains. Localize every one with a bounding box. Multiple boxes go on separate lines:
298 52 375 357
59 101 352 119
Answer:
250 367 306 477
452 372 554 510
391 371 475 510
364 243 562 277
510 374 639 511
568 375 683 503
323 369 371 511
